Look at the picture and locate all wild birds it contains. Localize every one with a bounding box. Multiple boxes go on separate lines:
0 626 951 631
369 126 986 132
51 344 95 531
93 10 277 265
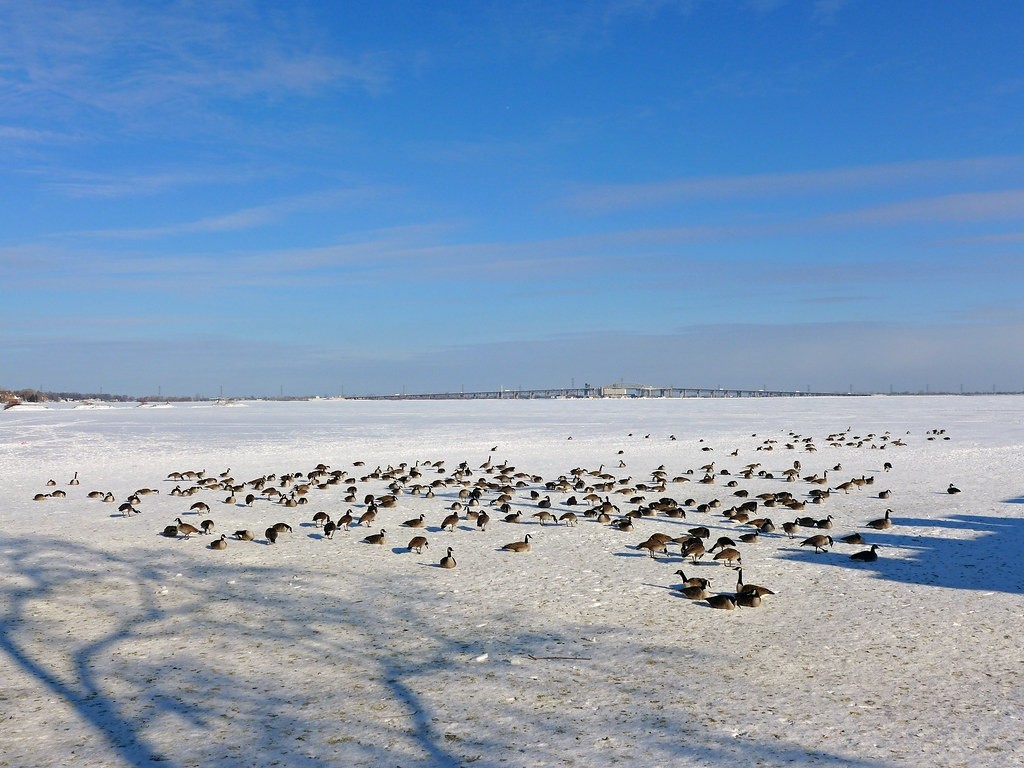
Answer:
33 426 961 610
